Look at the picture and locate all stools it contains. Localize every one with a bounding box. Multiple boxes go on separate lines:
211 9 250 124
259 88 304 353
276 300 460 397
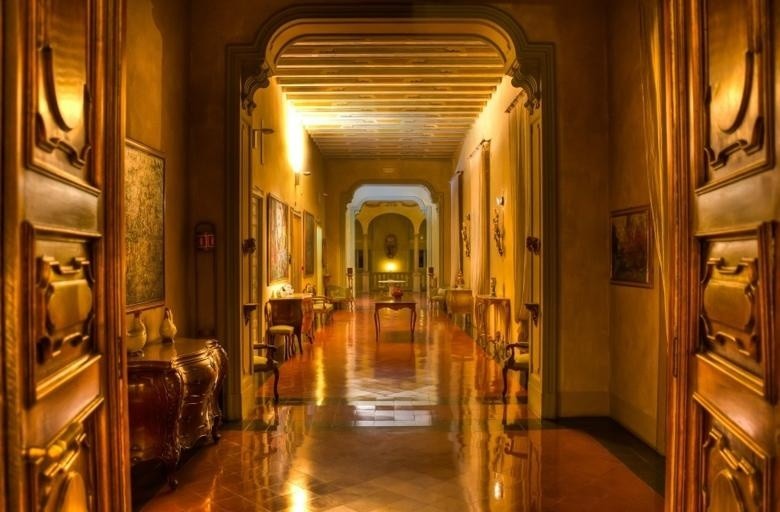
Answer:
268 325 294 360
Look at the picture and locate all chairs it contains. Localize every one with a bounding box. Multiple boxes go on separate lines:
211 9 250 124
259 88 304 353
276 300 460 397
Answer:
302 283 334 327
430 284 448 310
327 285 353 312
502 339 530 397
253 342 280 400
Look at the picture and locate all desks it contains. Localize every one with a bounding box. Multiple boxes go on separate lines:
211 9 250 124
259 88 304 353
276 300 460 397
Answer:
269 293 313 353
374 299 417 342
128 339 230 489
378 280 406 297
475 294 510 356
446 288 473 327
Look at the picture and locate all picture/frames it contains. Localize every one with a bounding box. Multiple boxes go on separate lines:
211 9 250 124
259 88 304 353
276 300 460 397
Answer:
268 191 290 283
608 203 654 288
303 209 315 276
124 136 167 315
